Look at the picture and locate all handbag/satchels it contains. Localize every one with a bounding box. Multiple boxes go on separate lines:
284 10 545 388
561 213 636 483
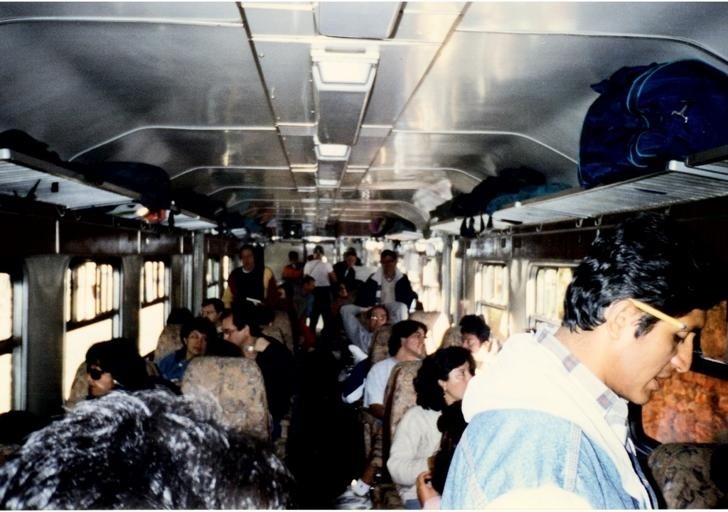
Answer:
576 58 727 191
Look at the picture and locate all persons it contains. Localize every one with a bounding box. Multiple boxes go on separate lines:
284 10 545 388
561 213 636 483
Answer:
439 214 727 508
0 234 502 509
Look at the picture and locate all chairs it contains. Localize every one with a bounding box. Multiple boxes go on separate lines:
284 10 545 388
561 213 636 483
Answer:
362 306 498 510
60 306 293 441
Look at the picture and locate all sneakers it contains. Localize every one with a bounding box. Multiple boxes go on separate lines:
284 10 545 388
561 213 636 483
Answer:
337 477 375 510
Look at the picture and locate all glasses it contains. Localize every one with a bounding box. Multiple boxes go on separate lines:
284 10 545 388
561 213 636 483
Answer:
85 365 108 381
370 314 388 321
